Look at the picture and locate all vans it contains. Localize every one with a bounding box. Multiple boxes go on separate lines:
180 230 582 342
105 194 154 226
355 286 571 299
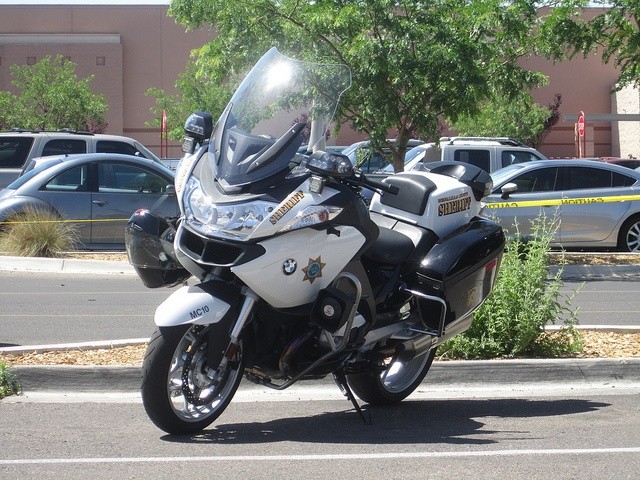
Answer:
0 128 166 194
341 138 426 177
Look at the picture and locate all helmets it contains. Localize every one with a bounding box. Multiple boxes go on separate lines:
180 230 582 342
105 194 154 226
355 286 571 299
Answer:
125 209 192 288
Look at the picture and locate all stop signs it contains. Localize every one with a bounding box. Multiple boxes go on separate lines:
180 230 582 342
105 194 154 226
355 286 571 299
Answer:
578 115 584 136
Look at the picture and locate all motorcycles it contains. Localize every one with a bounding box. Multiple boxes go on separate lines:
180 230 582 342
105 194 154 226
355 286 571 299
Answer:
125 46 506 434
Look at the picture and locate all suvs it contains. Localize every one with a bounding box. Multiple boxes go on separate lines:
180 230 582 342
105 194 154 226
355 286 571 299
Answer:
359 137 550 202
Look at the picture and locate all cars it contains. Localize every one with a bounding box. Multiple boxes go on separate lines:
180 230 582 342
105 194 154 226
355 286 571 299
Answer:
0 153 176 251
479 159 639 252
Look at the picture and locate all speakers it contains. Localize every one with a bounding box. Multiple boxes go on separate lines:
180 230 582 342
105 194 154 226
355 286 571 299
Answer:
309 288 355 333
